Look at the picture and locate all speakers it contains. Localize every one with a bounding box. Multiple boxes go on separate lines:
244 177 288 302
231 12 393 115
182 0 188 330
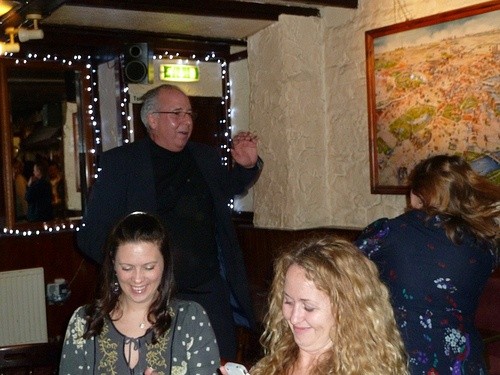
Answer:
121 42 154 85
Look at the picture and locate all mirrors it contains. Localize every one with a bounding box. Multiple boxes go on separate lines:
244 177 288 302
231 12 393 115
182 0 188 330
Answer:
0 59 96 235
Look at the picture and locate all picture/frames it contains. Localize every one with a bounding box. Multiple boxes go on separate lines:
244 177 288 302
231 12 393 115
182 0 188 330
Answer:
365 0 500 202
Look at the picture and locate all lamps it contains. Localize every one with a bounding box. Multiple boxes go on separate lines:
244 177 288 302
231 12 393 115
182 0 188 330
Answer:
19 13 43 42
0 28 21 56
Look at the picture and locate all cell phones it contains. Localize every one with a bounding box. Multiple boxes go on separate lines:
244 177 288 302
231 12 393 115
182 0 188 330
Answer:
225 362 249 375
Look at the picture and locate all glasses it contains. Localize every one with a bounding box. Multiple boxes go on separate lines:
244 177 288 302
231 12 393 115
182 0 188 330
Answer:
152 111 198 120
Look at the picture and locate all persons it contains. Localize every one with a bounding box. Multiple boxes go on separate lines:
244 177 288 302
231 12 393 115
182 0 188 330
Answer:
352 154 500 375
77 82 263 363
216 238 412 375
11 158 66 223
59 210 222 375
